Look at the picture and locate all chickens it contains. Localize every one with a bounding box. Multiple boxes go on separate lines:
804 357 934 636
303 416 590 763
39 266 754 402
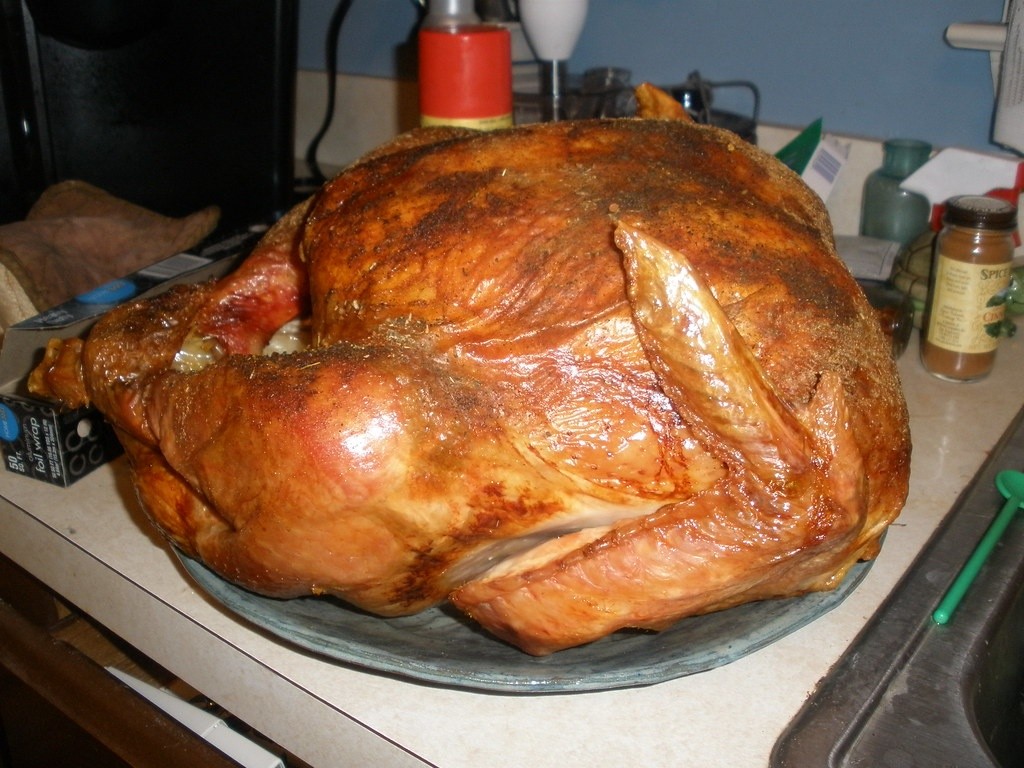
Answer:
23 80 914 659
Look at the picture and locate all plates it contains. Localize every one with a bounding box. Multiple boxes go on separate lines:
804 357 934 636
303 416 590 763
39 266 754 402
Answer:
165 527 890 695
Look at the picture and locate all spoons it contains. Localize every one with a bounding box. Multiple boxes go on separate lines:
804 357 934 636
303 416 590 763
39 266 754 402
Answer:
934 468 1024 625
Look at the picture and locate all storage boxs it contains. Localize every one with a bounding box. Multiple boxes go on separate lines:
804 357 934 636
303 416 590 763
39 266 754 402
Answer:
0 216 273 490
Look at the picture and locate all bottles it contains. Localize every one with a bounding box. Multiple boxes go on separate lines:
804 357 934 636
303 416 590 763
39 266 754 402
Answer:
922 195 1016 383
859 135 932 245
